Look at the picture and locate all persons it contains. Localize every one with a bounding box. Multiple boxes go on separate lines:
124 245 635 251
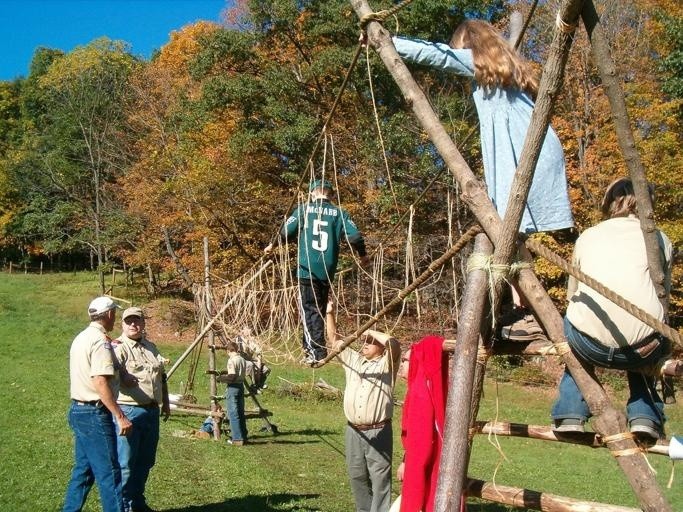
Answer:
354 17 577 311
325 297 401 512
236 327 270 395
216 342 249 447
111 307 170 512
188 403 222 440
264 180 370 365
551 176 674 442
58 297 133 512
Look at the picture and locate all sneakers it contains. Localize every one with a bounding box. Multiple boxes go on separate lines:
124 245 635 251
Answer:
261 384 268 389
502 314 550 341
629 419 666 441
551 419 585 434
249 384 263 395
553 228 578 242
227 440 247 445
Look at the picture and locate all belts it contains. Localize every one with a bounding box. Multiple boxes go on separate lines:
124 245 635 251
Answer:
579 332 658 353
348 420 391 431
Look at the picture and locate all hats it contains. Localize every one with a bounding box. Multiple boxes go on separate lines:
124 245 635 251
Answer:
88 297 125 316
309 180 333 191
123 307 144 319
